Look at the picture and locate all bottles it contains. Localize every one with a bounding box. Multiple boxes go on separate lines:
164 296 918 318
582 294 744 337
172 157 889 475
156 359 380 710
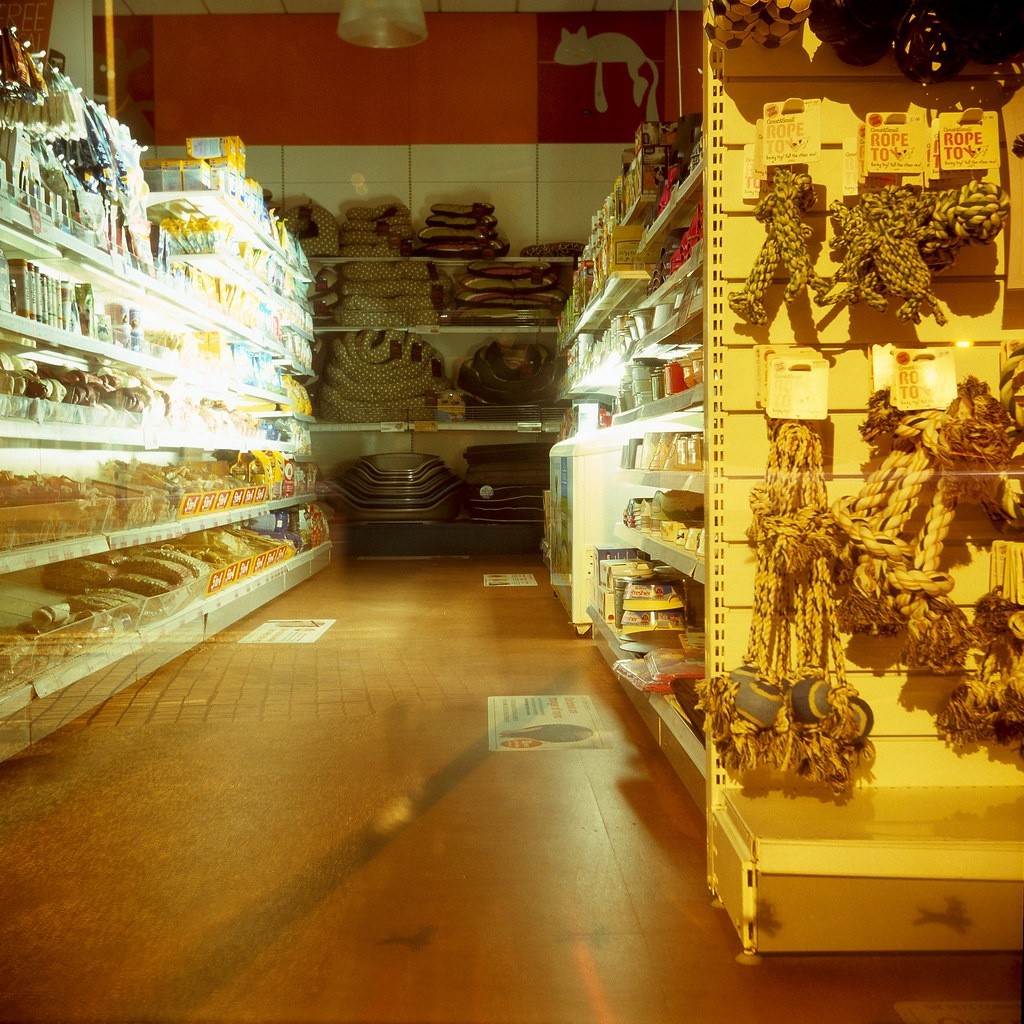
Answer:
651 346 704 401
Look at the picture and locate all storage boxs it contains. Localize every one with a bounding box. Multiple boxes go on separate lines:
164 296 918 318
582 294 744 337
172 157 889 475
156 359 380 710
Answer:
141 135 272 232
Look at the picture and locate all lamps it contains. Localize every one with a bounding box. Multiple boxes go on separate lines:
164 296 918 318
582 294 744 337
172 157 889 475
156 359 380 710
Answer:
331 0 438 53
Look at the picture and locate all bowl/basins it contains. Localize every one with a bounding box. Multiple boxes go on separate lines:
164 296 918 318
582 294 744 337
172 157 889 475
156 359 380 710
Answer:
611 357 668 414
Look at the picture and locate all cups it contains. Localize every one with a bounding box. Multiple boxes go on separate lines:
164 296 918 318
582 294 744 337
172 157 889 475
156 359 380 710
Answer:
620 432 706 473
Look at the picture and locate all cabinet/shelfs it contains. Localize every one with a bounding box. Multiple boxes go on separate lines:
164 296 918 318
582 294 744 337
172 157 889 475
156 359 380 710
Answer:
531 157 713 829
0 187 332 722
281 176 569 565
